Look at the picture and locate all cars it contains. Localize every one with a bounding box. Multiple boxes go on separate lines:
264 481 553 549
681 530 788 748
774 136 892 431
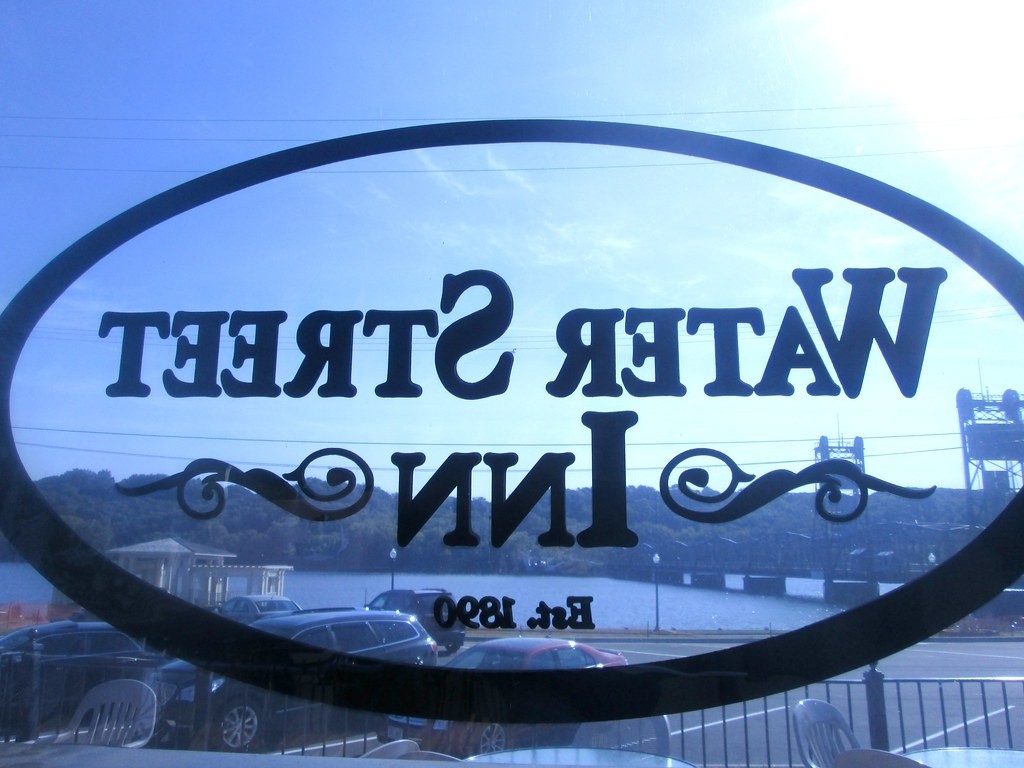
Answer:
213 594 302 626
0 606 356 744
375 637 629 760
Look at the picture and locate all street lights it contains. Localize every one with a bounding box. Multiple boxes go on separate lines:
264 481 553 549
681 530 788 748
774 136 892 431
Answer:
390 547 397 589
652 552 661 630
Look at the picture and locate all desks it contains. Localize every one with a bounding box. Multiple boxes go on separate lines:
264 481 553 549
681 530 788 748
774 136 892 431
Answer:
464 746 698 768
900 747 1024 768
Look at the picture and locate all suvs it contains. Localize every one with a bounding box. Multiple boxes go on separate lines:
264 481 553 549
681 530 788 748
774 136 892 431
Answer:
152 606 438 755
363 586 466 652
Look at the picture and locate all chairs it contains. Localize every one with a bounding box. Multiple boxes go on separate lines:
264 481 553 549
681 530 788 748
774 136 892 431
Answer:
360 699 929 768
51 677 157 749
